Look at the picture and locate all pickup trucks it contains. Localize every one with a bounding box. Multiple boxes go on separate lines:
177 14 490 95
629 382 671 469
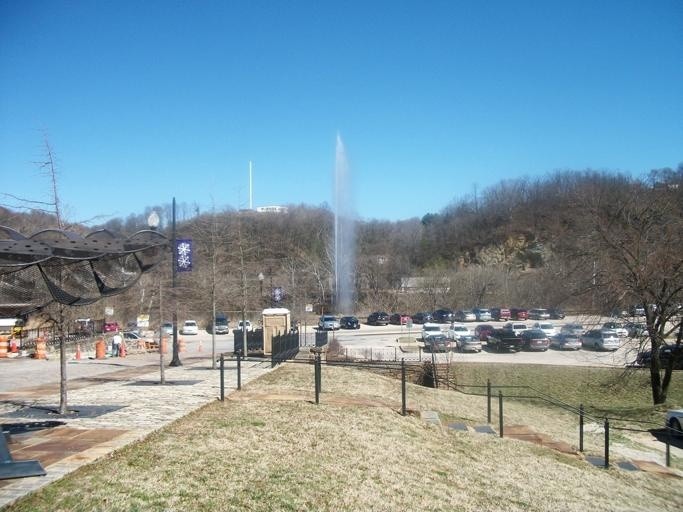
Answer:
484 328 523 353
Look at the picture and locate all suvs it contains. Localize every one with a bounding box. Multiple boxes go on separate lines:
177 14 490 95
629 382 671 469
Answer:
317 315 341 330
420 322 442 341
580 328 619 351
120 330 155 349
72 318 91 334
503 321 528 336
181 319 198 336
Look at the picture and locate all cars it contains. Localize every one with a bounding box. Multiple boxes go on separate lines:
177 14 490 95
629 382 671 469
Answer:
475 325 495 341
560 323 586 336
365 305 565 327
236 320 253 332
514 330 551 351
422 333 451 352
664 408 683 436
339 316 360 329
454 334 482 352
160 321 173 335
603 301 682 367
549 332 583 350
531 322 555 337
448 324 470 340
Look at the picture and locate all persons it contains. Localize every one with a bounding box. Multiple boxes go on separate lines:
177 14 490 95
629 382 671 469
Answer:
109 335 122 358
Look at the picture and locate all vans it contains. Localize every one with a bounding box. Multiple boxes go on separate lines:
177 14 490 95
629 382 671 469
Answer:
210 312 230 334
104 320 119 332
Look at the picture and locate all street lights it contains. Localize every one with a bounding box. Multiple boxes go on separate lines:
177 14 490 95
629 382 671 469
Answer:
257 265 283 309
146 196 183 367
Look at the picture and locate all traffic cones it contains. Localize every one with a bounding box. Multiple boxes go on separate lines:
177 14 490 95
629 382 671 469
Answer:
197 340 203 353
9 334 19 353
119 341 126 358
74 344 81 361
